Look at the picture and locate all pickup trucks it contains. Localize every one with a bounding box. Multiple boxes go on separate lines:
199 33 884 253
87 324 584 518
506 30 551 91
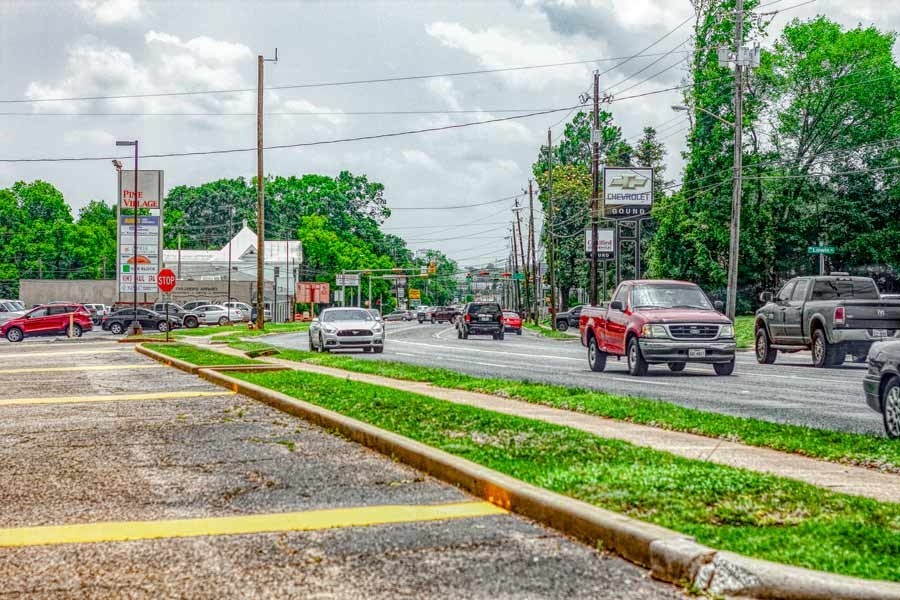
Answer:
578 279 737 378
753 271 900 368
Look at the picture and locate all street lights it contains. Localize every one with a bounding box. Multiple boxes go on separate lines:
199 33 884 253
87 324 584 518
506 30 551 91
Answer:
115 139 138 311
512 206 557 332
670 103 743 321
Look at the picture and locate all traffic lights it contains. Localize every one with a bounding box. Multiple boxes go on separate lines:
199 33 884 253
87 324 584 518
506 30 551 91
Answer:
501 273 512 276
480 272 489 275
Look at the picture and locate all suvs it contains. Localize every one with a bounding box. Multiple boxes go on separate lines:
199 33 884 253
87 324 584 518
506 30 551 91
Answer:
383 301 524 341
0 303 94 343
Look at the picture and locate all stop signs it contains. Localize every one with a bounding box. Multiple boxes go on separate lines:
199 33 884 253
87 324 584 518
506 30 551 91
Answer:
156 269 176 292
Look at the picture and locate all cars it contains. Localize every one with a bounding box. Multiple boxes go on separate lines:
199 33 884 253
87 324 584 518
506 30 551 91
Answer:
0 299 273 335
308 306 386 354
863 336 900 440
550 305 583 332
